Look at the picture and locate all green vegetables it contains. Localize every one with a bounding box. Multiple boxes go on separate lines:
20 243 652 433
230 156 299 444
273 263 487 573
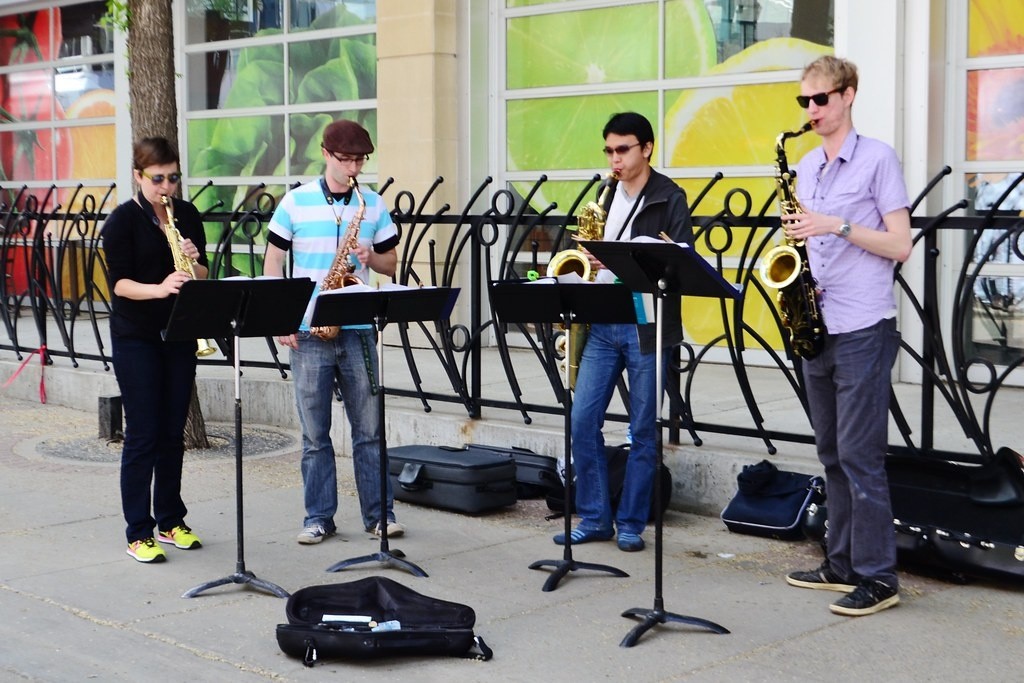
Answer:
186 4 377 276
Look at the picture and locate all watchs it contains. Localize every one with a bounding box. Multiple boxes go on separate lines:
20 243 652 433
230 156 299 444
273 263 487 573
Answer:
838 219 851 239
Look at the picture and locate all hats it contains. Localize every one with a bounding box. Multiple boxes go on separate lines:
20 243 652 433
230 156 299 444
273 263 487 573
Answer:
322 120 375 156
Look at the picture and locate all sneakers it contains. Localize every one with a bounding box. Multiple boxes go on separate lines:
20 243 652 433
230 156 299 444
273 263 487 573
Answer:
366 517 404 541
126 536 165 564
297 521 330 543
831 576 900 616
158 525 202 548
786 560 858 593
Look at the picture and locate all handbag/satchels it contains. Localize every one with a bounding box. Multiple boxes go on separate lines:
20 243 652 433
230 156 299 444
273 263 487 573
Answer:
600 438 672 519
718 459 824 537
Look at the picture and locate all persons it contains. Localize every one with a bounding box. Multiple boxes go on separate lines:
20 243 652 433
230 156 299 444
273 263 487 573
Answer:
786 57 914 617
103 136 210 562
264 119 405 544
553 112 695 552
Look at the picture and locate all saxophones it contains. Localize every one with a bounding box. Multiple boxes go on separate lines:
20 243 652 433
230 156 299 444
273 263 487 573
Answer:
757 119 829 361
159 193 220 359
308 177 365 340
544 168 621 388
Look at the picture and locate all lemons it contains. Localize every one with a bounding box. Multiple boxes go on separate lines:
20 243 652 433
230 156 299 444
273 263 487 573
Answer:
501 1 837 350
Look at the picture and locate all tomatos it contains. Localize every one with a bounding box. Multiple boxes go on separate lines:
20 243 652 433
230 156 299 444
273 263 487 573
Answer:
0 7 73 299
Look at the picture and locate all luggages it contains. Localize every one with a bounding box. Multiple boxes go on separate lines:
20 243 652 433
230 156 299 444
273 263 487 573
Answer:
384 437 517 513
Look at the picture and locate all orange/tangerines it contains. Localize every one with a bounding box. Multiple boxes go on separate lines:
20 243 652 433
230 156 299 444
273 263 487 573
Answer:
64 88 117 238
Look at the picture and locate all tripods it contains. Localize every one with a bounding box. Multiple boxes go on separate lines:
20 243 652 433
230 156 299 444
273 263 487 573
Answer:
578 240 743 647
310 288 462 577
489 284 639 592
159 278 313 598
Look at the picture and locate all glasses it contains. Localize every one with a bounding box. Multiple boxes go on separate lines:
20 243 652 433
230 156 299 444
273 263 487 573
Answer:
330 151 369 166
795 84 845 108
602 139 648 156
137 168 183 184
